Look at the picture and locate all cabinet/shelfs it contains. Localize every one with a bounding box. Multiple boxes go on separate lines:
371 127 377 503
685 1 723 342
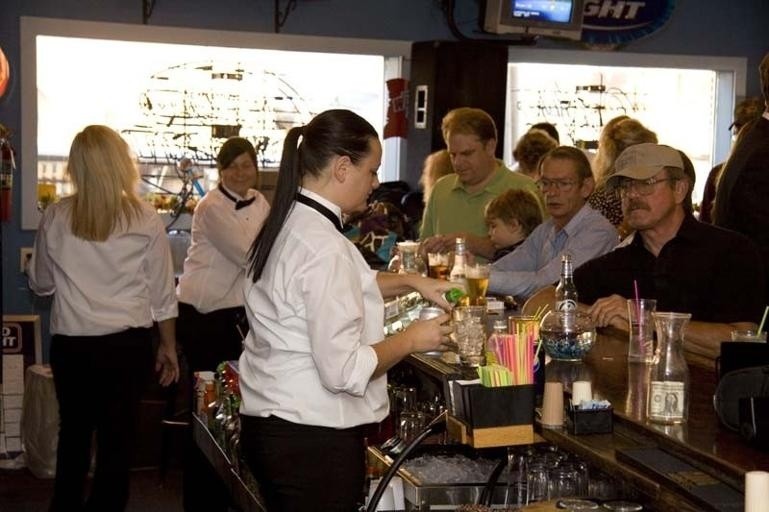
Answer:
195 348 697 511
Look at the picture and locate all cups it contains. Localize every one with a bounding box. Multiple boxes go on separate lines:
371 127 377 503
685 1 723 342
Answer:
540 382 566 428
626 300 660 361
727 329 769 343
463 261 490 304
427 252 450 280
397 241 421 270
626 362 656 419
452 307 489 365
572 381 594 405
507 445 645 512
743 471 769 510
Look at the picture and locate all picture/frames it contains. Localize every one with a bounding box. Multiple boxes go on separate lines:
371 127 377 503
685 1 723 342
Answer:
0 315 43 470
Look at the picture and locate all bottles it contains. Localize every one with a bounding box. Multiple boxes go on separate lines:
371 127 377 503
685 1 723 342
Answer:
447 236 469 285
645 312 695 424
385 382 446 444
554 254 579 312
206 364 244 485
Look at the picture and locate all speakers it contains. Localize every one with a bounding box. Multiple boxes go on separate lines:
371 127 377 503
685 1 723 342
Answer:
406 39 506 196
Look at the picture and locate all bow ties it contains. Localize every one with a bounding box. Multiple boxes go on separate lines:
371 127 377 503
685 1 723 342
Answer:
235 196 256 210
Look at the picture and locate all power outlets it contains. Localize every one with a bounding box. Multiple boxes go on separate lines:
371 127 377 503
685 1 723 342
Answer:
20 246 35 273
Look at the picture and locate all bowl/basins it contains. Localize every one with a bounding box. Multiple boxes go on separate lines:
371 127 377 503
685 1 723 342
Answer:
211 124 241 138
539 311 597 362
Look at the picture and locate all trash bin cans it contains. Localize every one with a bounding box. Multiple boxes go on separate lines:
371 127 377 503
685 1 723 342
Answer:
25 364 97 480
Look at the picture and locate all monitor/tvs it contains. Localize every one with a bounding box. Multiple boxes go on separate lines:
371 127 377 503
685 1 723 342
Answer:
483 0 585 41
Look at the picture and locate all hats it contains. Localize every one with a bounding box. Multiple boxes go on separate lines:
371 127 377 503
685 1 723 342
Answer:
728 96 765 130
607 143 695 187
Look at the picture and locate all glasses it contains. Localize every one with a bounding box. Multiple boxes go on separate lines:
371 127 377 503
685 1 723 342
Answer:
536 174 584 193
615 177 680 196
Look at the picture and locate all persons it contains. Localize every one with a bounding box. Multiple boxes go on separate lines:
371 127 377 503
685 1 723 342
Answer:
699 54 769 234
161 137 286 511
413 108 769 330
28 126 183 511
243 106 454 511
